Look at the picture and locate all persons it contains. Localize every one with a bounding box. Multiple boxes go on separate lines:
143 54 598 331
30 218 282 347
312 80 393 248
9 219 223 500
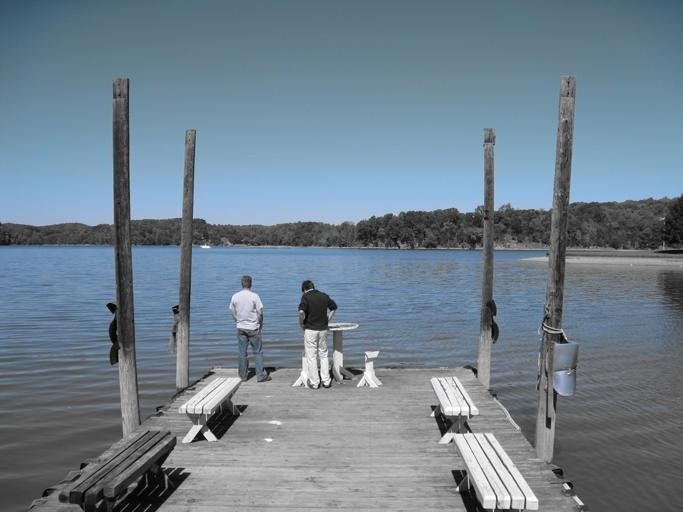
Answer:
228 275 272 382
298 279 338 390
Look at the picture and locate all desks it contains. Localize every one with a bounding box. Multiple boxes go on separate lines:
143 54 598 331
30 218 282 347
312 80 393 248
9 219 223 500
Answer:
328 323 359 384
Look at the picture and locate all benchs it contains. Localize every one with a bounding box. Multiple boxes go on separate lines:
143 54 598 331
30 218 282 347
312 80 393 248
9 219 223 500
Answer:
454 432 539 511
179 376 243 443
59 428 176 512
430 377 479 444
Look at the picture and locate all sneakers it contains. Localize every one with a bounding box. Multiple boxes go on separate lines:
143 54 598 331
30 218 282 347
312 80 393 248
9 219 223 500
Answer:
241 370 254 381
257 375 271 383
321 375 332 388
306 377 318 389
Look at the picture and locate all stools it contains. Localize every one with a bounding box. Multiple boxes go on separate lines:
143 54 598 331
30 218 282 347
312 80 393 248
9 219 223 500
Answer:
357 351 383 388
